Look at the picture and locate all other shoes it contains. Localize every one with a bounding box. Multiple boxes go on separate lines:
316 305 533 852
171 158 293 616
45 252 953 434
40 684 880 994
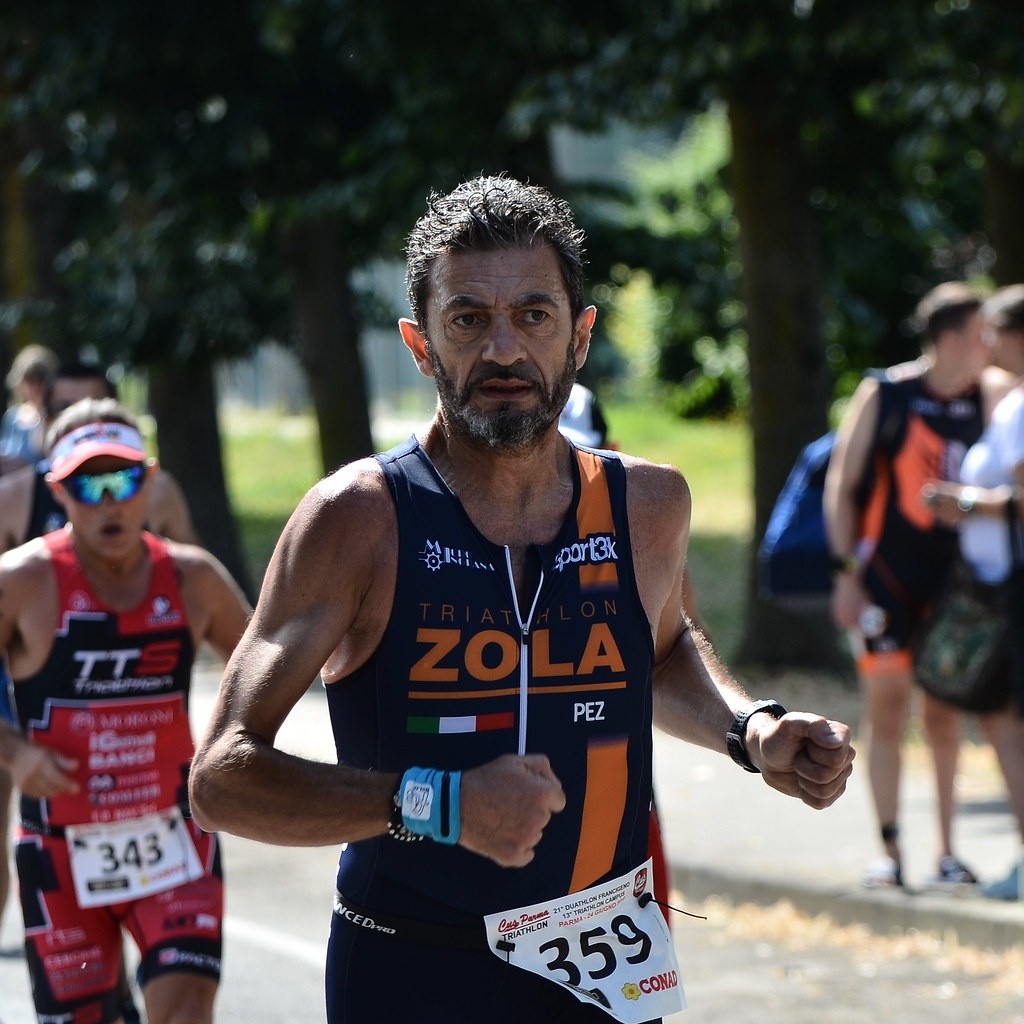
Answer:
924 855 980 887
980 865 1019 899
861 858 903 890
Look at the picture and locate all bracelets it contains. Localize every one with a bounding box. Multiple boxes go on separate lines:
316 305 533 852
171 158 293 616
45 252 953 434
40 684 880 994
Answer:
386 766 462 844
959 491 977 513
832 560 854 576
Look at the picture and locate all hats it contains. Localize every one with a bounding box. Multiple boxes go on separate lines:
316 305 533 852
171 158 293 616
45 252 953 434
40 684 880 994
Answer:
49 423 147 481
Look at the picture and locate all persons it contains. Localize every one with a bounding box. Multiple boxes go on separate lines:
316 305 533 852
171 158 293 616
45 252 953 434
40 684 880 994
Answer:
186 169 856 1024
1 397 254 1023
822 283 1017 888
559 385 670 928
919 282 1024 898
0 363 196 553
0 345 58 459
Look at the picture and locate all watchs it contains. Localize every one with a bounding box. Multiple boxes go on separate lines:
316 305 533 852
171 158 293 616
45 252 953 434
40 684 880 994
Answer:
726 698 787 774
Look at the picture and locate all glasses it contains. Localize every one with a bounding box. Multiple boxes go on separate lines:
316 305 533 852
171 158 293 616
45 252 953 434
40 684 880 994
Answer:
58 463 146 506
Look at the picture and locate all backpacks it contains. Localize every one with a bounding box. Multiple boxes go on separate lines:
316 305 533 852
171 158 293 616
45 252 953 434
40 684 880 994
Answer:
760 367 907 598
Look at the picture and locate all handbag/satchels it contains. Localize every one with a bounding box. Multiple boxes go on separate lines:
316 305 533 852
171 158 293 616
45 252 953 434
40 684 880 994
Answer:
916 578 1021 713
